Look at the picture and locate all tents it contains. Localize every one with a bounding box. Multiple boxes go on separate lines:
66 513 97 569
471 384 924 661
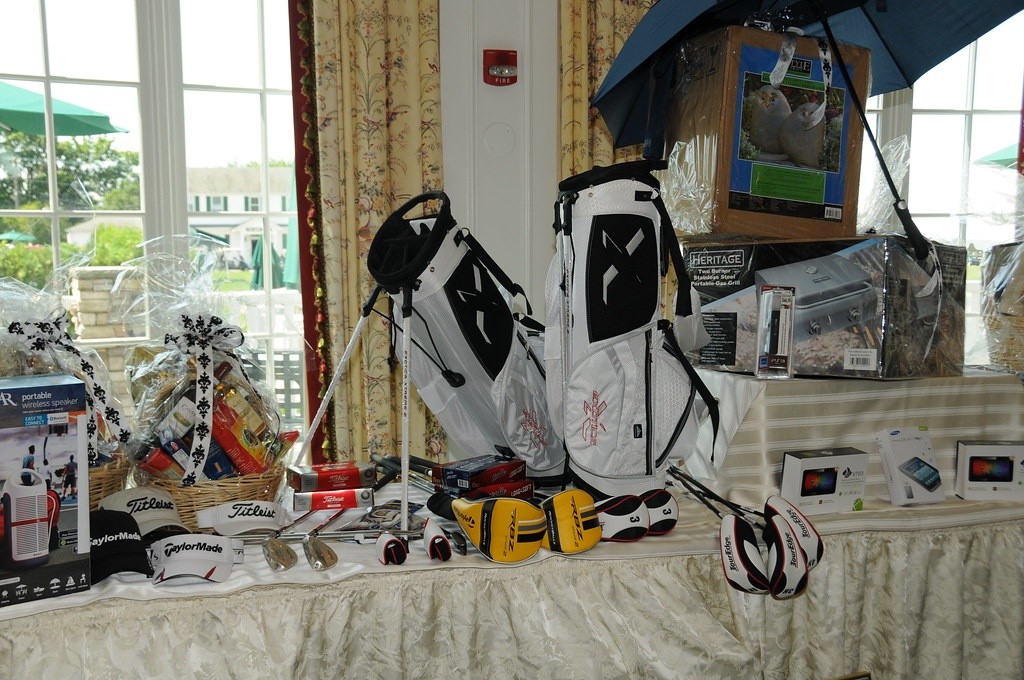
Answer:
282 219 302 296
251 234 282 288
289 162 297 210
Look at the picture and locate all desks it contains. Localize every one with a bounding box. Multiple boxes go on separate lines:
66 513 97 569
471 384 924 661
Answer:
0 484 1024 680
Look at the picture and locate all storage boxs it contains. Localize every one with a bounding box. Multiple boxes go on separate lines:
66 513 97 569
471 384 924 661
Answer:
953 440 1024 499
434 480 534 507
285 460 377 491
666 234 968 385
430 454 526 489
292 489 375 511
0 371 92 608
872 425 947 507
779 446 870 515
644 25 872 247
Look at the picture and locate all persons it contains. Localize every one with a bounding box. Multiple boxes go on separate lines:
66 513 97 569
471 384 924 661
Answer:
39 459 53 489
60 455 78 501
21 445 35 486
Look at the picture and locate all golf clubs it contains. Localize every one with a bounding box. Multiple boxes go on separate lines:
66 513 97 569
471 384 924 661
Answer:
302 471 398 572
228 529 470 558
261 510 319 573
667 465 825 602
369 451 679 564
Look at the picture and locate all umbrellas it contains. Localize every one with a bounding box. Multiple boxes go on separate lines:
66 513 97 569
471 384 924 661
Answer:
588 0 1024 261
0 83 130 134
0 229 36 242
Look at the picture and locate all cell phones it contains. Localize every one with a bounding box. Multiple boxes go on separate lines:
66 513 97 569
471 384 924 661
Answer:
899 456 941 491
969 456 1014 483
801 467 837 497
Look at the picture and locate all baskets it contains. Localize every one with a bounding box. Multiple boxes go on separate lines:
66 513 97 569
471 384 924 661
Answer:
133 458 285 533
88 460 128 513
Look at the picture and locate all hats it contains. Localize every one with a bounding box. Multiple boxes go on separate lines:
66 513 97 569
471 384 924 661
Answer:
97 485 193 537
150 533 243 584
90 506 154 585
